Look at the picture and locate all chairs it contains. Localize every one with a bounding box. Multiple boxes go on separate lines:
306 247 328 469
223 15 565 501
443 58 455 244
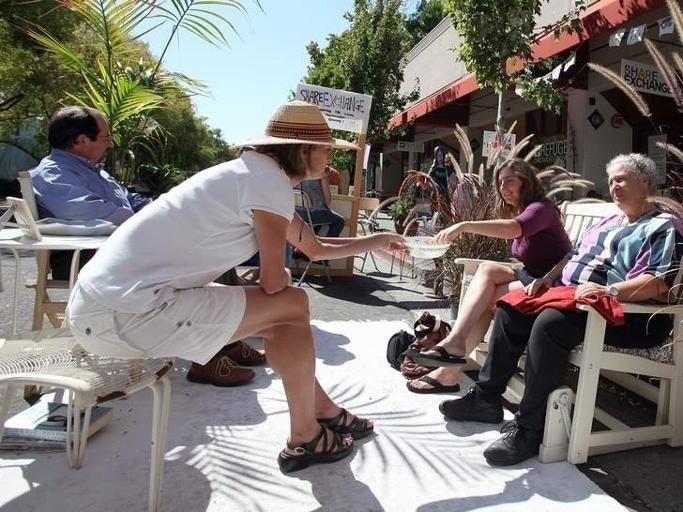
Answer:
0 337 176 512
17 171 77 332
352 220 378 272
356 197 379 235
389 217 426 280
292 189 334 286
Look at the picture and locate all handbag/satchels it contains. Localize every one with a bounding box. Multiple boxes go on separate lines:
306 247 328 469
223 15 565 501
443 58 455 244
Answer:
387 330 416 370
399 311 452 376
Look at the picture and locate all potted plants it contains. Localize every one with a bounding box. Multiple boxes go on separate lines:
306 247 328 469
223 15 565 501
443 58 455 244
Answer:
416 177 512 327
386 180 420 237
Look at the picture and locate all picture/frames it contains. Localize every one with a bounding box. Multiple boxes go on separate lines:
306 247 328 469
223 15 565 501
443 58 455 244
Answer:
0 196 42 241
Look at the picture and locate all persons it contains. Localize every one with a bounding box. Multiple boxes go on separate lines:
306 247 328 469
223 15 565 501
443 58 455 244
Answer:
406 158 573 392
329 166 343 194
64 102 408 474
29 107 266 388
294 166 346 265
439 151 682 465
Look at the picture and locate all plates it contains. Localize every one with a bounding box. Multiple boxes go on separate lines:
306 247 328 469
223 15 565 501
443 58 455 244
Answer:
397 237 452 258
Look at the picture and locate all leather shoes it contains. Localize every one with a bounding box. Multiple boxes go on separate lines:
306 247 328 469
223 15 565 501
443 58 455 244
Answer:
217 341 266 366
187 354 256 387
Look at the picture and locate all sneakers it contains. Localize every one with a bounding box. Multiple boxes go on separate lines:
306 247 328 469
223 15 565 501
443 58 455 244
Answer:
439 386 504 423
483 422 539 466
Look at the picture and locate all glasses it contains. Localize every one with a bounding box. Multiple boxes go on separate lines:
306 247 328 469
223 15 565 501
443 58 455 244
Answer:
98 135 113 144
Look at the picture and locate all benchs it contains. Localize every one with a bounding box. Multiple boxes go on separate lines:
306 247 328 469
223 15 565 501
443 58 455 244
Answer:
453 199 682 465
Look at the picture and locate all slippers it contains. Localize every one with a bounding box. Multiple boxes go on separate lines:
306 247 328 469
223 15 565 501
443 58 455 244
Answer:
412 345 467 368
292 251 303 260
320 260 329 265
406 375 460 394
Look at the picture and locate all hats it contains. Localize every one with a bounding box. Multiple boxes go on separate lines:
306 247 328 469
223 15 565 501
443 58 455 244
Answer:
234 99 363 151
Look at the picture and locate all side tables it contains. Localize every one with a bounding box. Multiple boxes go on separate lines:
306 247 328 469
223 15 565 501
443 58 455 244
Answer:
0 227 110 402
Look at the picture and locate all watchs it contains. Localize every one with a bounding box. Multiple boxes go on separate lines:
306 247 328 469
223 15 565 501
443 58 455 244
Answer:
608 283 622 302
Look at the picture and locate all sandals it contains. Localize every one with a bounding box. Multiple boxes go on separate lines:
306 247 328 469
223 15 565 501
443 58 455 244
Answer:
278 423 354 473
317 408 374 440
